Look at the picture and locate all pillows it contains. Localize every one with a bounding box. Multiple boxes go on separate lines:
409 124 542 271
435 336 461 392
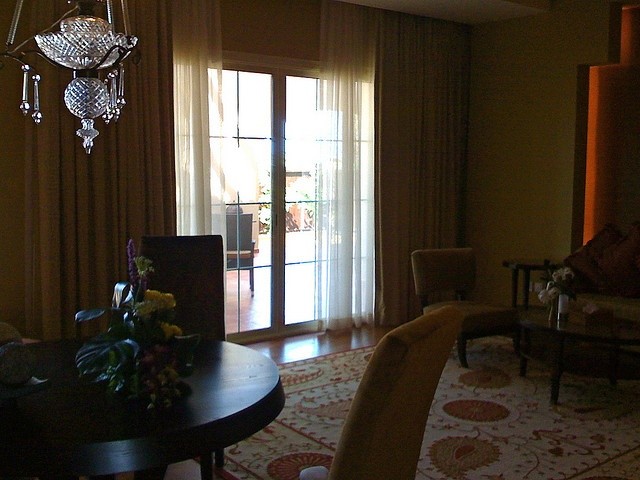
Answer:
564 222 625 294
593 220 639 298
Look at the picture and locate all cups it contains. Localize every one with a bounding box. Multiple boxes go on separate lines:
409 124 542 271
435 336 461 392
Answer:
558 295 569 322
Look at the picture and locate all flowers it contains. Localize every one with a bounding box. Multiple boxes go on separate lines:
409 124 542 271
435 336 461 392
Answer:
538 267 575 304
74 239 201 412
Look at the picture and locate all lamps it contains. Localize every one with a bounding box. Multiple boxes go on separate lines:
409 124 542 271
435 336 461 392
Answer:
0 0 138 155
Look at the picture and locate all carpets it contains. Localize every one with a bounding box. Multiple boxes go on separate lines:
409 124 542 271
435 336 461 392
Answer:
192 334 639 480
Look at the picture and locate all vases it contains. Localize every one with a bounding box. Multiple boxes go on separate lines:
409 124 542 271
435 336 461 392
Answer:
549 301 557 320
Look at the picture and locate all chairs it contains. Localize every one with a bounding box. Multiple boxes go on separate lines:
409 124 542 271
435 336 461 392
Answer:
227 214 256 298
139 235 225 480
328 305 465 480
411 247 520 367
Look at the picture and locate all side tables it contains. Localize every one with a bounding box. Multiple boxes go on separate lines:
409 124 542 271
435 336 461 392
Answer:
503 259 556 308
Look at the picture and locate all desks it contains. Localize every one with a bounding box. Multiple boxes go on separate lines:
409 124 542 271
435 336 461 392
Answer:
0 337 285 480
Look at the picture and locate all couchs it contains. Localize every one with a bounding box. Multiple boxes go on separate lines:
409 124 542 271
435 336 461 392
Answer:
576 284 640 353
